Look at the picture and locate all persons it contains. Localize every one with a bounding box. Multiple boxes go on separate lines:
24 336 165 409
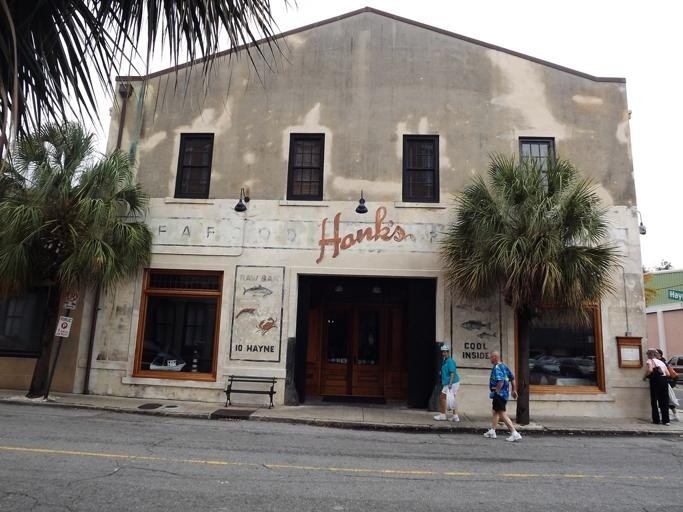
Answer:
483 350 523 441
641 347 670 425
656 349 680 420
432 343 460 422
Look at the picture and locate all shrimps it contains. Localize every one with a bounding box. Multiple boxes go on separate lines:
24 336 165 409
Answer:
235 308 259 320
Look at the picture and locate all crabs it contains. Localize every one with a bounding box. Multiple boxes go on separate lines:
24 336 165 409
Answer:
255 317 279 337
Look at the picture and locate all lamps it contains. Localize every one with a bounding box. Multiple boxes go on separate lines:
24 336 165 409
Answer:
354 189 368 215
232 188 250 212
636 211 646 235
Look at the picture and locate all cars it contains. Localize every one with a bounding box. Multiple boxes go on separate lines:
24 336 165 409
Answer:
527 351 596 376
141 345 190 372
666 354 682 382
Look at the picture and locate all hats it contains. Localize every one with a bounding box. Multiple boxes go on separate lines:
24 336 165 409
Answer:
440 345 448 351
646 348 656 354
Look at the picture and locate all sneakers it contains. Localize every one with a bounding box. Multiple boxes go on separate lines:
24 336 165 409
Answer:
433 415 446 420
505 434 521 441
670 416 679 421
448 417 459 421
483 430 495 438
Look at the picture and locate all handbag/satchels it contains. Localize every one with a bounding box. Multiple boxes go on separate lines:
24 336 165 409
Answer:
647 366 663 375
667 365 678 379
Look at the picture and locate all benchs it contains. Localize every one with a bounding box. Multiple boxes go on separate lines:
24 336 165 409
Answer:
222 374 277 409
